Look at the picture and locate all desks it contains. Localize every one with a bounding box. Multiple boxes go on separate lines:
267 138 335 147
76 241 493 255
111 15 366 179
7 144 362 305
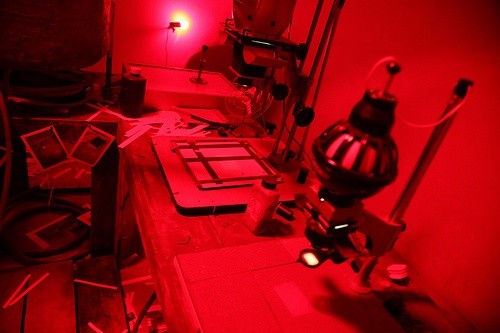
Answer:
109 62 489 331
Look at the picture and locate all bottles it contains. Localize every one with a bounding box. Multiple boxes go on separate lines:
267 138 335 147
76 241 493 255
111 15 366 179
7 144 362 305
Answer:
242 177 280 236
119 67 145 118
